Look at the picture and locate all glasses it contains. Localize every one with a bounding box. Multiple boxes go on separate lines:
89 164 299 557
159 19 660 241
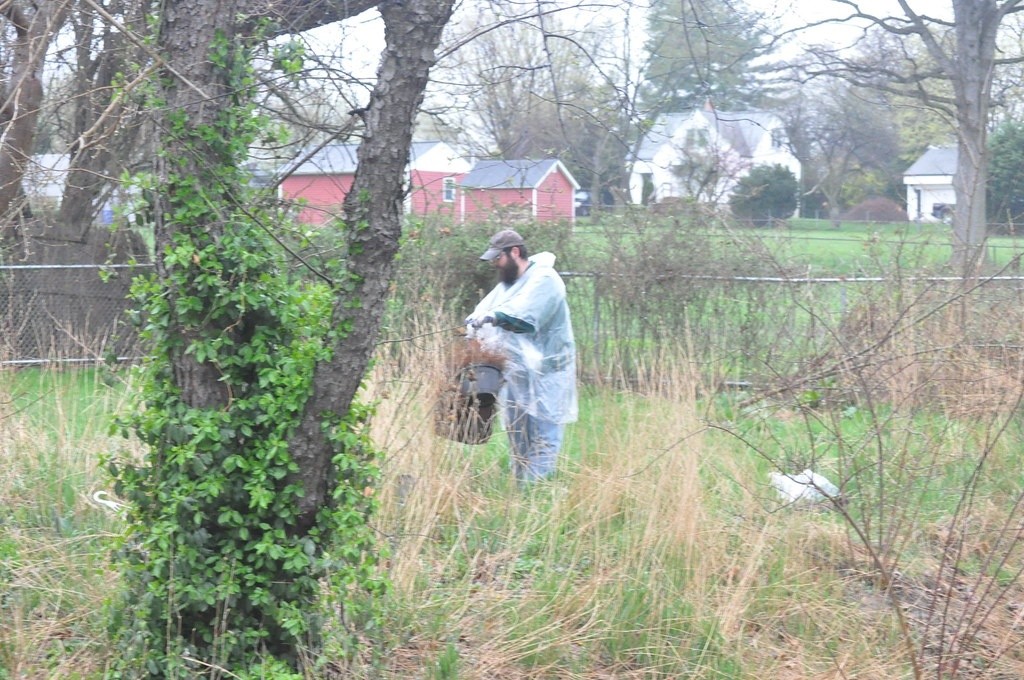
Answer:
488 252 506 264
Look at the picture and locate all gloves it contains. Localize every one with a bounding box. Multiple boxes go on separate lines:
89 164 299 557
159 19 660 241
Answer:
463 310 497 328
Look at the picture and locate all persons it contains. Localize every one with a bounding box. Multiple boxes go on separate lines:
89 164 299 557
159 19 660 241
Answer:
603 187 614 214
465 228 571 492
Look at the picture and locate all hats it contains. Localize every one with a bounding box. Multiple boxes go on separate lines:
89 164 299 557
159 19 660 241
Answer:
479 230 525 261
